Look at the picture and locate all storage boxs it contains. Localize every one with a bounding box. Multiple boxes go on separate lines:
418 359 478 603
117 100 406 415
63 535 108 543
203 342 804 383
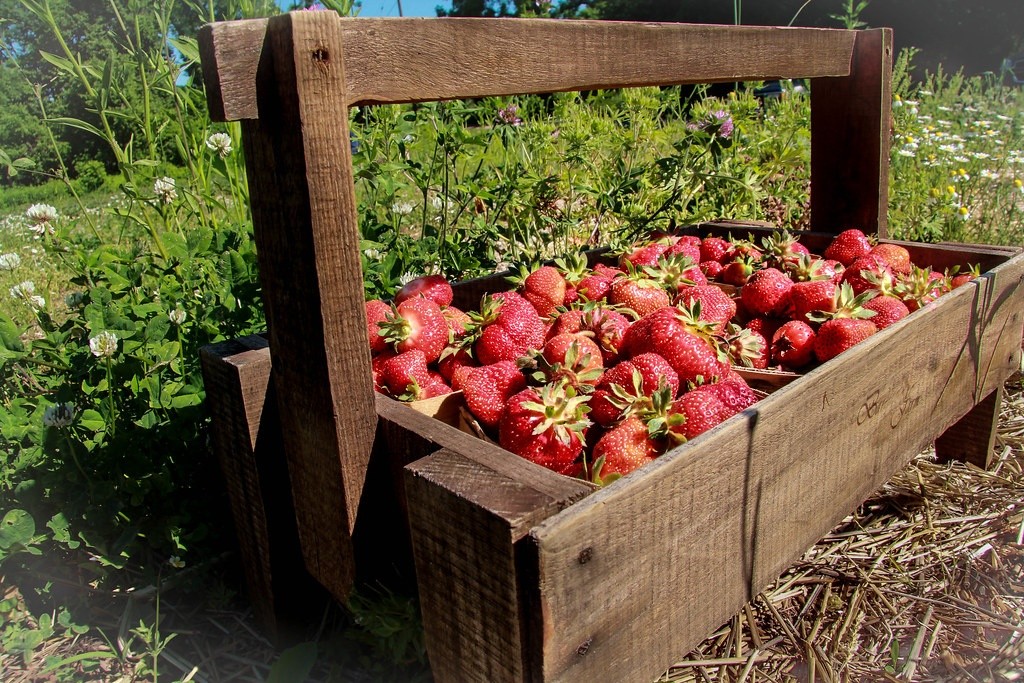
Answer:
196 10 1024 683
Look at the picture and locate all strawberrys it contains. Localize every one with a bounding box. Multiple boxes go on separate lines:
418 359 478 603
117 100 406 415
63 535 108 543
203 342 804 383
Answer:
365 228 985 489
